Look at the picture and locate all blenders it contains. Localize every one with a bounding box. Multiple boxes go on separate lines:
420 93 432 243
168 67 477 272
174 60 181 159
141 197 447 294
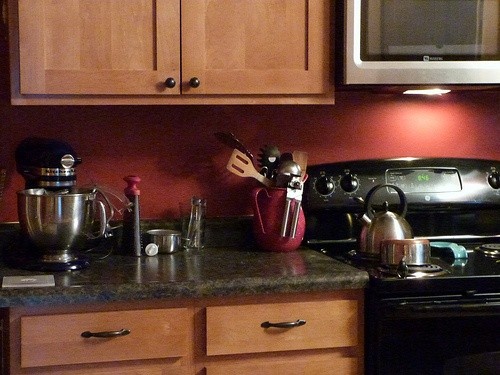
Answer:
15 135 95 271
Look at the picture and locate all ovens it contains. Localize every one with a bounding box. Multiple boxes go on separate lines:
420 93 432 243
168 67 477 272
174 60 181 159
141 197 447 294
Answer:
364 293 500 375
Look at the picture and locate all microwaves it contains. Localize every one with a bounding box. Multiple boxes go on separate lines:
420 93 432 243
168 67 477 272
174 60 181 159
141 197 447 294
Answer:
341 0 500 85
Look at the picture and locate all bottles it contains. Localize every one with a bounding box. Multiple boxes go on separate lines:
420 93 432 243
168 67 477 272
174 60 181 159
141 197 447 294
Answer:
186 197 207 250
121 175 144 258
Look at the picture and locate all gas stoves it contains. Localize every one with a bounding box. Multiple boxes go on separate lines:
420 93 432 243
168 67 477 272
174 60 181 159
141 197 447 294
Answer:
301 157 500 291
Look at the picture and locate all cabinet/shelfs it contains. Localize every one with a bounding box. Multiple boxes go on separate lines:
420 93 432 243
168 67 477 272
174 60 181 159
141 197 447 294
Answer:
8 289 365 375
7 0 335 103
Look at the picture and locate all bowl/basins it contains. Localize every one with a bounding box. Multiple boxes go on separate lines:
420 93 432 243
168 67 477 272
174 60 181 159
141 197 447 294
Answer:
17 187 106 248
146 228 182 254
380 234 432 265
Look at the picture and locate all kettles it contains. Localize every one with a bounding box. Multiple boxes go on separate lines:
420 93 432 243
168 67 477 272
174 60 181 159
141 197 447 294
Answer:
344 184 414 257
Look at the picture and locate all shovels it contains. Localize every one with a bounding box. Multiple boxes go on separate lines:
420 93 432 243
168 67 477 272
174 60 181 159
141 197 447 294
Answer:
226 148 273 188
215 130 252 163
292 151 307 181
